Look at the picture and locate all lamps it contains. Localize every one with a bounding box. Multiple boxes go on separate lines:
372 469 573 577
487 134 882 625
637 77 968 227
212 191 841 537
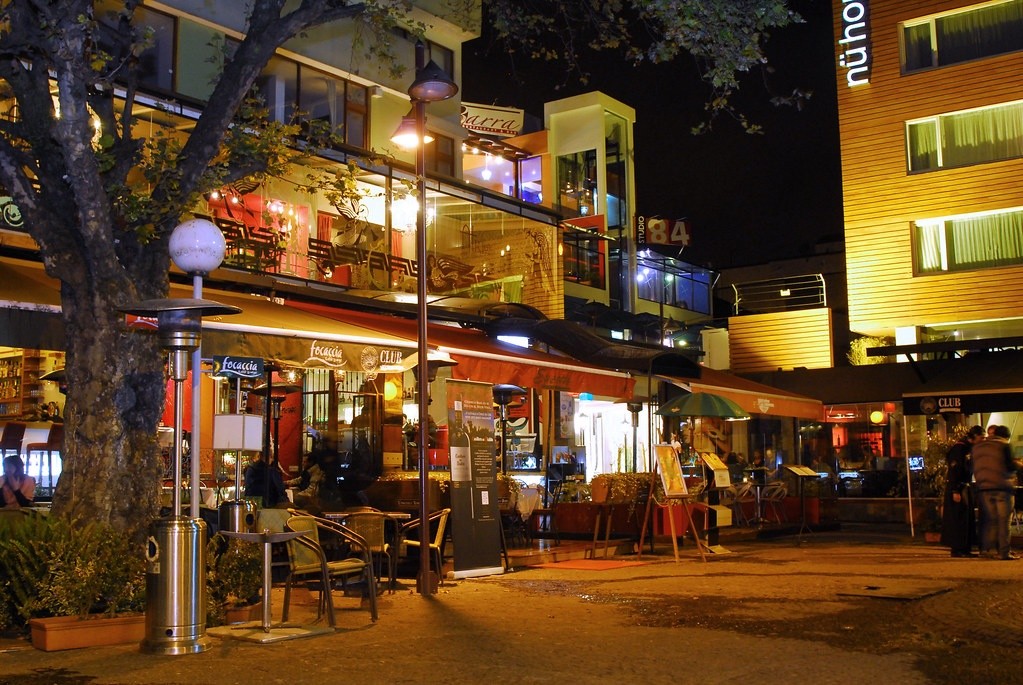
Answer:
169 219 227 277
389 108 436 148
407 41 459 103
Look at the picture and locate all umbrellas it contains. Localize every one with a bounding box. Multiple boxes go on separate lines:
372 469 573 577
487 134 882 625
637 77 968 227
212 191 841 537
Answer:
654 392 750 446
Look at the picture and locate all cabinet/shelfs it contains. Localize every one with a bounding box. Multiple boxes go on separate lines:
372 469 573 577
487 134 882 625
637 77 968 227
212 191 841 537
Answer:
0 355 47 441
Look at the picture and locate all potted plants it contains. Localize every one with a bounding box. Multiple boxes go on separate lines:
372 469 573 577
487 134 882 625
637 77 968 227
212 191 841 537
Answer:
28 529 146 652
216 537 263 625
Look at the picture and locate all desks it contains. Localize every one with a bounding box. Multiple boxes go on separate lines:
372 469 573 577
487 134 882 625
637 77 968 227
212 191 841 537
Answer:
321 512 411 594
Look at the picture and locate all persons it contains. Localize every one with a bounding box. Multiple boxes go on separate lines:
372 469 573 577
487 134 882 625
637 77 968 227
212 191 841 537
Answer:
718 447 780 481
939 422 1018 560
861 447 875 469
242 449 326 515
1 455 35 509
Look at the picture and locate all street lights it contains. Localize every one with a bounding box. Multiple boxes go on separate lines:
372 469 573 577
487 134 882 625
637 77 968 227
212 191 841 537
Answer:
386 36 461 600
169 217 230 522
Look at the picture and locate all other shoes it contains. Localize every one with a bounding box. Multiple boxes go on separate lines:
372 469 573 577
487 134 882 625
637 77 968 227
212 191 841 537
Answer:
998 553 1021 560
951 552 978 558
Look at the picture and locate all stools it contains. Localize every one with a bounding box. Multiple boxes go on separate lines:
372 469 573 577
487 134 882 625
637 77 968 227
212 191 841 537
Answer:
0 422 64 497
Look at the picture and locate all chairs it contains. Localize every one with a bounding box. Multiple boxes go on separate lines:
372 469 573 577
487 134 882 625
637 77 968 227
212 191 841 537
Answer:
500 491 518 548
282 506 452 628
525 478 563 547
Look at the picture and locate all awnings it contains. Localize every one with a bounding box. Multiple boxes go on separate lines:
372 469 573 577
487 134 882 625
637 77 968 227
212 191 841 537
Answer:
653 363 822 419
0 262 637 399
